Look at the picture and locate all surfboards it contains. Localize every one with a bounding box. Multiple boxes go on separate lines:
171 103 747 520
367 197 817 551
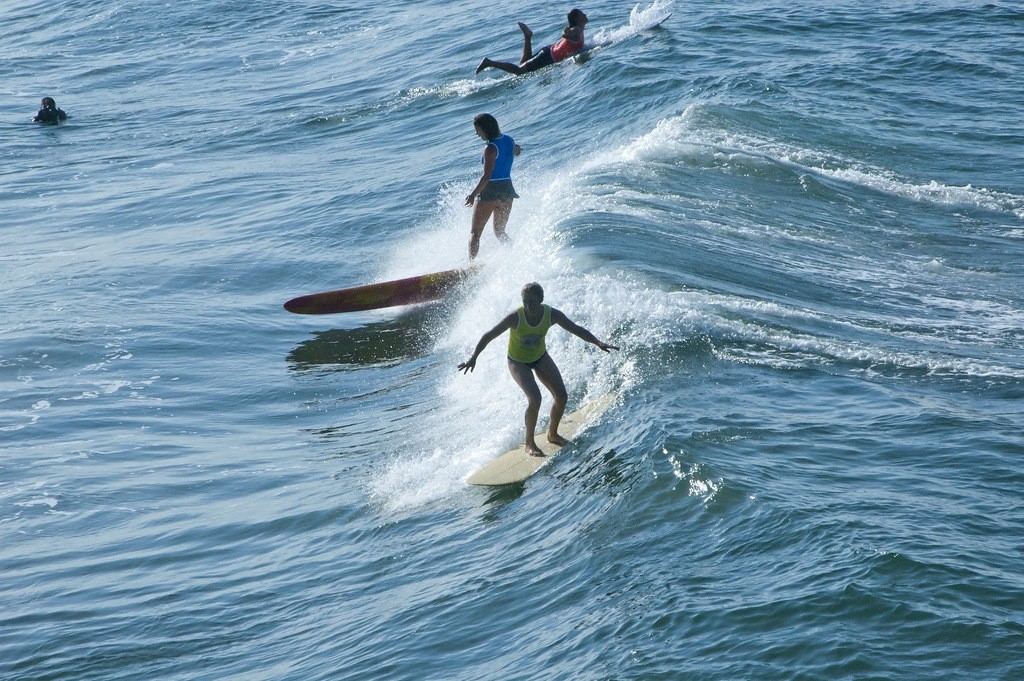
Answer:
463 389 618 487
470 11 672 91
282 264 482 314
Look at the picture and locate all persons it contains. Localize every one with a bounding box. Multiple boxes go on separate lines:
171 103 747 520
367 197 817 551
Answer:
458 281 621 457
463 112 522 263
32 97 65 122
475 9 589 75
